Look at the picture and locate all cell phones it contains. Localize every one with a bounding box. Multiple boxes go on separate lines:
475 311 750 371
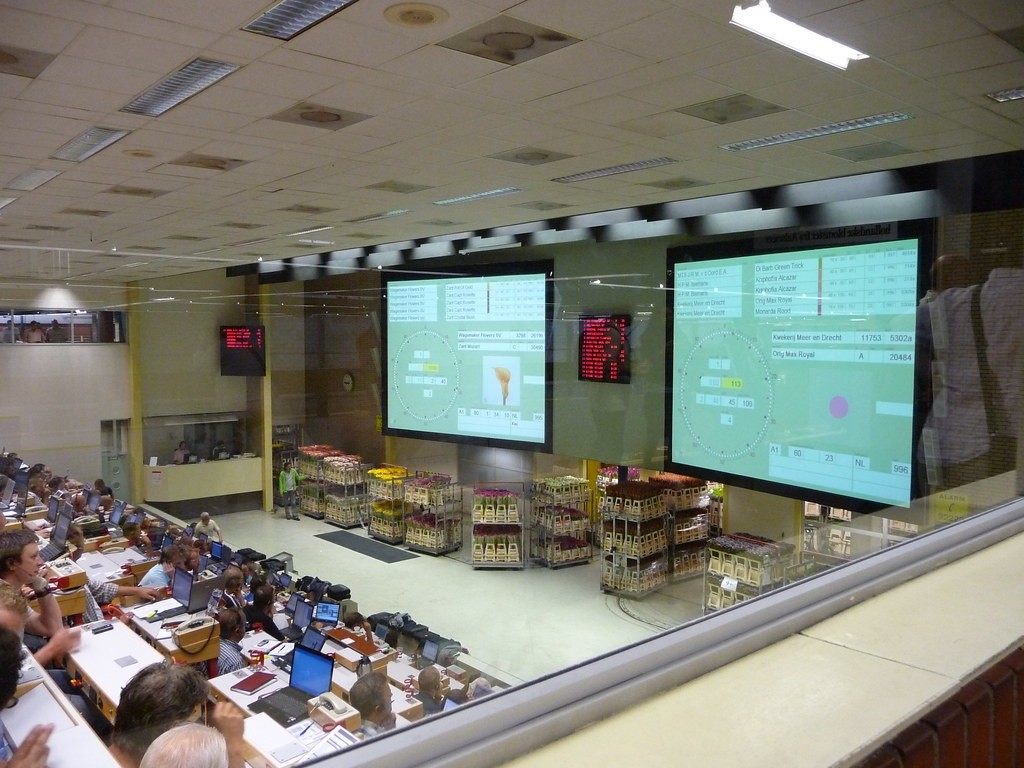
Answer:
257 640 269 647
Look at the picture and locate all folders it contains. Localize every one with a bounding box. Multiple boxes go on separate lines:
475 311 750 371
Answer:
248 639 295 657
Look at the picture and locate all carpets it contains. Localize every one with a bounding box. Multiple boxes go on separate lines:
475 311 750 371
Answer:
313 530 420 563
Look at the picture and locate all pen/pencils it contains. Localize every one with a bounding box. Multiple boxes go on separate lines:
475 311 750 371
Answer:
300 721 314 735
278 645 285 653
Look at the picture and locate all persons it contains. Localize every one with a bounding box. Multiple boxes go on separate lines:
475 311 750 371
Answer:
174 442 190 463
0 453 373 675
109 662 245 768
1 320 67 343
350 673 396 739
441 689 469 710
0 627 56 768
279 462 308 521
212 441 226 455
416 666 441 714
466 677 491 699
139 723 229 768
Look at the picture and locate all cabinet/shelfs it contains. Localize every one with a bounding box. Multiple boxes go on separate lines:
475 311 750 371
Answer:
472 481 525 569
530 478 594 569
272 445 464 555
595 467 795 615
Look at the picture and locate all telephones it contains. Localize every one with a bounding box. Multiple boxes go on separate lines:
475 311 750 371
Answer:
25 505 49 519
72 515 100 532
175 615 222 647
98 538 129 553
307 691 362 734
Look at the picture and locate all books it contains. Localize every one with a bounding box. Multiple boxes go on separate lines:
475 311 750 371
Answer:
231 671 277 696
248 639 295 657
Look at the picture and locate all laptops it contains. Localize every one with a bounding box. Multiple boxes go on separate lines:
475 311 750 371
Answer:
0 456 440 728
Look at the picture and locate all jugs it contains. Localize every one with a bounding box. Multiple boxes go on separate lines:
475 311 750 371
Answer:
355 657 372 679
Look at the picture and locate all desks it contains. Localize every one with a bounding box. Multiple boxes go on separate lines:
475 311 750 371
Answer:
0 489 464 766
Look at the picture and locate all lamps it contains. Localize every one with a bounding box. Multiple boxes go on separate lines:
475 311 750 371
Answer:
729 0 870 71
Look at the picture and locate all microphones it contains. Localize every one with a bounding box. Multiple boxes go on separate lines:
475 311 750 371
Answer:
13 563 33 577
